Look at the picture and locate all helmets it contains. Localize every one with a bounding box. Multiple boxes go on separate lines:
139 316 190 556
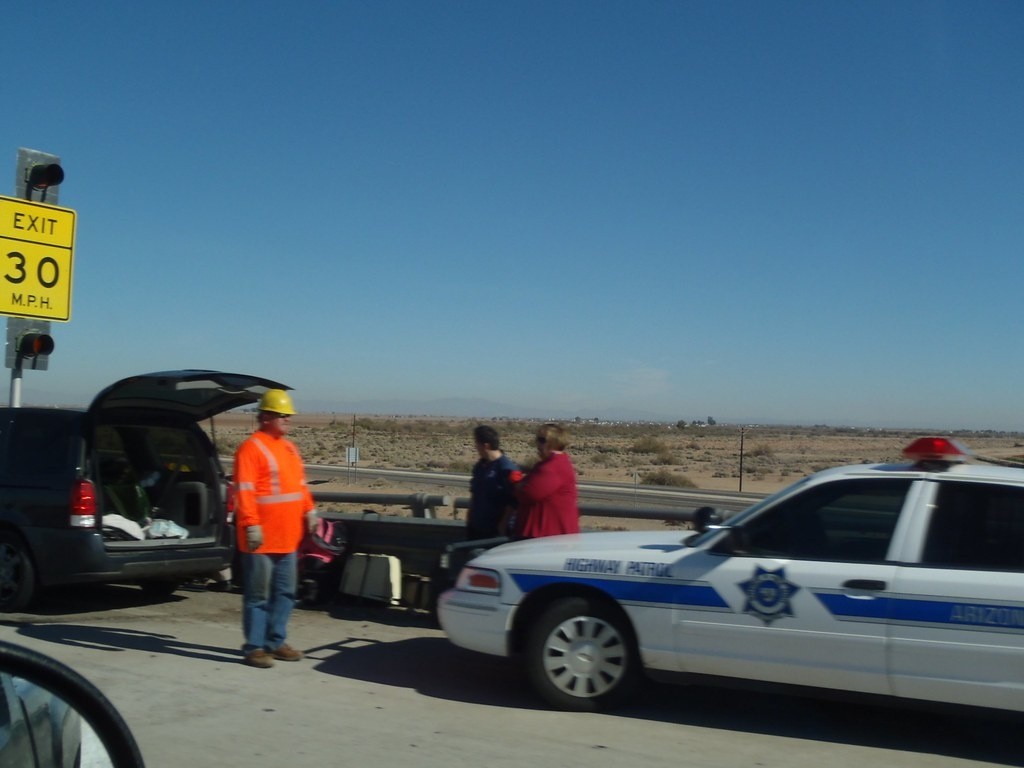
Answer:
258 388 297 415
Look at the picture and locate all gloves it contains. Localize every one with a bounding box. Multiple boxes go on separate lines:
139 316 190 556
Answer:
305 509 318 533
246 524 262 551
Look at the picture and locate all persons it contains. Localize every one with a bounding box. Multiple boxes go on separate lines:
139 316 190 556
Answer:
462 425 521 539
233 389 321 669
512 424 581 539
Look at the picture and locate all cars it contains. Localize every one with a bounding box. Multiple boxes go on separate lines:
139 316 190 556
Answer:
432 437 1023 722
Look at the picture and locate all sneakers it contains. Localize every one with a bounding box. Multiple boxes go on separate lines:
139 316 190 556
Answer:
242 643 271 668
267 644 300 662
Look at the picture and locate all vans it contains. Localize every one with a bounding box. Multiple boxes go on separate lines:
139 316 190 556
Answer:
0 368 298 612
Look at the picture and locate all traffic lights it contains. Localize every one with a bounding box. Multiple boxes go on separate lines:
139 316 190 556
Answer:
19 331 56 359
31 163 66 191
